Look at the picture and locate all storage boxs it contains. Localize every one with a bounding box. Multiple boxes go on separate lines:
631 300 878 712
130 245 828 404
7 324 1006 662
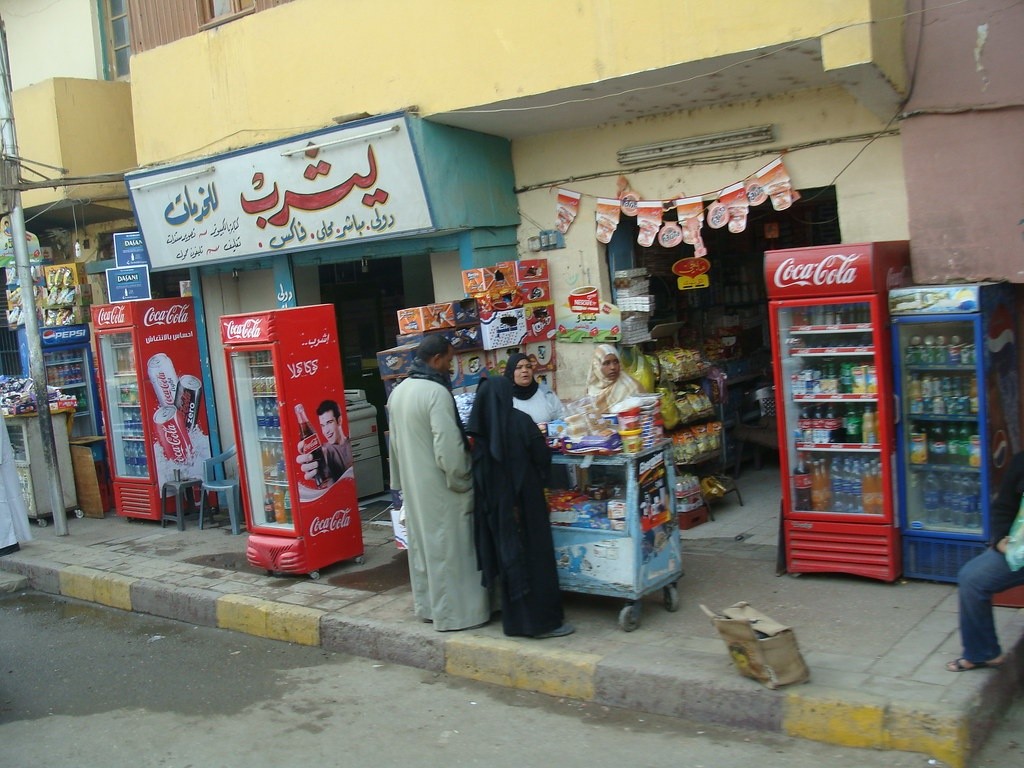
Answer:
105 231 152 304
700 600 809 690
376 258 557 456
44 262 93 324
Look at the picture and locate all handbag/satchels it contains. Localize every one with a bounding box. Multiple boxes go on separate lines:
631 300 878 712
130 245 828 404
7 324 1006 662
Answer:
702 600 810 690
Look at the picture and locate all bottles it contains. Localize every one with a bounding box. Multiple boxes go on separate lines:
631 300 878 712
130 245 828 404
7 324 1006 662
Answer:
798 403 878 444
265 486 292 523
919 471 982 527
911 421 980 466
47 363 82 386
294 403 335 490
261 442 287 481
124 442 149 477
256 398 281 438
793 451 881 514
123 408 142 436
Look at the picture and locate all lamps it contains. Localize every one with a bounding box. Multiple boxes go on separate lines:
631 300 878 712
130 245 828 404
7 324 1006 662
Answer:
617 123 774 167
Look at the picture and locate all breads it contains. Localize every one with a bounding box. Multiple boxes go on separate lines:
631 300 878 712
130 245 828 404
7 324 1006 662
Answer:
564 405 616 438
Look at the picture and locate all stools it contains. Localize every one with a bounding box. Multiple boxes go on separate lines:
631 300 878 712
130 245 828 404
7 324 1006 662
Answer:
161 477 215 532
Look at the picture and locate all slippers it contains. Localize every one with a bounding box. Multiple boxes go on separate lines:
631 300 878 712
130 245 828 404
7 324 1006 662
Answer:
533 623 574 638
945 652 1006 672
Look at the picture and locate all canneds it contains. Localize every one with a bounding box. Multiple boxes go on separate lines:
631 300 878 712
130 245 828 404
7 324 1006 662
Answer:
153 404 194 465
250 351 277 396
905 334 978 416
147 353 179 407
799 302 869 325
791 369 839 394
174 375 202 433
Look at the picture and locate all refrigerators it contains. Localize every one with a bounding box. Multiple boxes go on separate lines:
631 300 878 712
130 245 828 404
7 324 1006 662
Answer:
91 297 217 525
17 323 104 461
889 279 1019 584
763 240 914 582
219 303 365 579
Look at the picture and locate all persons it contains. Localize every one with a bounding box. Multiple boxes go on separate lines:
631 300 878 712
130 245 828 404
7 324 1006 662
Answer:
944 449 1024 672
503 353 571 491
465 375 576 639
386 334 500 632
296 400 353 490
0 412 31 558
584 345 645 416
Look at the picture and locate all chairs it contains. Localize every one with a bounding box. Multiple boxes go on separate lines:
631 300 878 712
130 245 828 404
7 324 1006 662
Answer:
742 387 775 471
199 443 241 535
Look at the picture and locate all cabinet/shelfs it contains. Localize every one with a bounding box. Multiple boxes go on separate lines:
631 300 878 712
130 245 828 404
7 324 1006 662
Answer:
4 407 84 527
544 438 684 631
341 390 385 499
607 221 776 482
